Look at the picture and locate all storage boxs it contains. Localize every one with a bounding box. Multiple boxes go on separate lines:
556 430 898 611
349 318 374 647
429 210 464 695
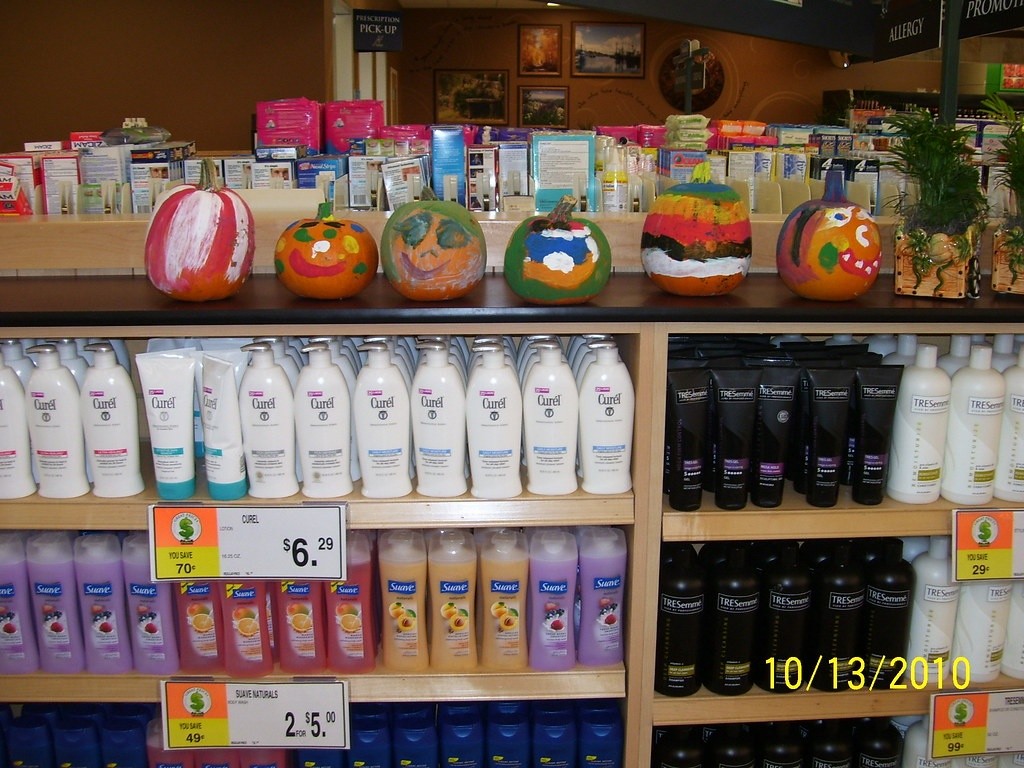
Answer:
0 99 469 218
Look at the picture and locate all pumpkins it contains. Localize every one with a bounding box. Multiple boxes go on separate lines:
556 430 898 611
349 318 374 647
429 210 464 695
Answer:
144 157 256 301
380 186 487 301
274 201 379 300
775 169 882 301
641 161 753 296
503 195 612 306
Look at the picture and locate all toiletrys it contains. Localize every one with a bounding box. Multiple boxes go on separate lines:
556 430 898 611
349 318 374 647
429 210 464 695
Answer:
0 338 1024 768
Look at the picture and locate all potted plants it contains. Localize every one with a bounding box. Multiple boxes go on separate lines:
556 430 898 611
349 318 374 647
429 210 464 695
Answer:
874 104 990 298
985 93 1024 300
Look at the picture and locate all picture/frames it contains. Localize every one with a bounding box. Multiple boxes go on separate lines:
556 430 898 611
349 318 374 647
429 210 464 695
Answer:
518 85 570 130
570 21 644 79
431 67 510 125
515 22 562 78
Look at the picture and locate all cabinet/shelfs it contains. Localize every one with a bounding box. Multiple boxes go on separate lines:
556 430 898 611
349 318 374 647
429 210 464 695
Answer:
0 321 1024 768
